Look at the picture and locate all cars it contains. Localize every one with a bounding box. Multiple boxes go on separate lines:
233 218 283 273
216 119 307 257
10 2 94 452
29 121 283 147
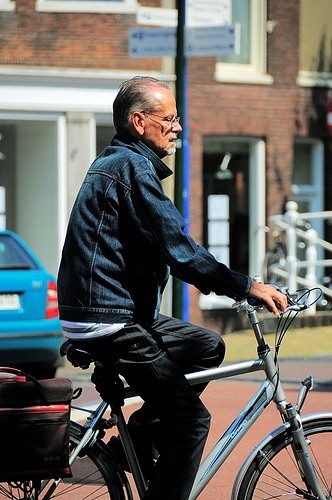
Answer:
0 230 66 378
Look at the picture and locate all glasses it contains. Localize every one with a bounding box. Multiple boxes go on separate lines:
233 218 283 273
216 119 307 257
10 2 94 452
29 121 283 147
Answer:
138 109 180 129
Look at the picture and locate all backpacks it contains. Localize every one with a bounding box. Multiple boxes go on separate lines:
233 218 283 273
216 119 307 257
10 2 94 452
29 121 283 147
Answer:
0 378 73 482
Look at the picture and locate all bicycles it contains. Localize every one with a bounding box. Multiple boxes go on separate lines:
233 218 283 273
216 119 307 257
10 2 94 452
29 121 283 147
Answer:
0 274 332 500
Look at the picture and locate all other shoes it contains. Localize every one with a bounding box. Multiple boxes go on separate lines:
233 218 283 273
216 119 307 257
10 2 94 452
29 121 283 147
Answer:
106 436 162 478
